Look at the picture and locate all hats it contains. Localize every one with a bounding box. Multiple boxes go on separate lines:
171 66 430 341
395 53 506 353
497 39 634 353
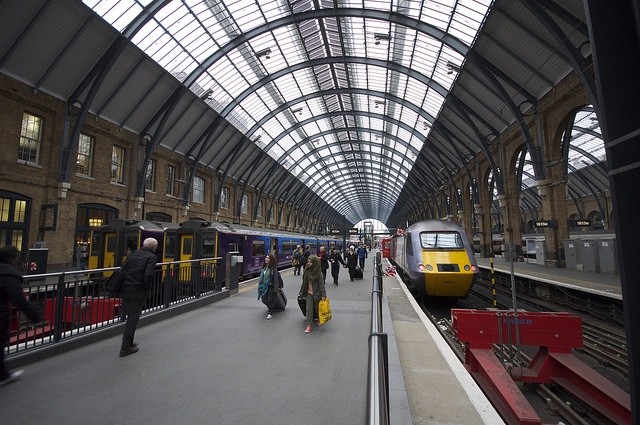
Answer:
350 245 355 250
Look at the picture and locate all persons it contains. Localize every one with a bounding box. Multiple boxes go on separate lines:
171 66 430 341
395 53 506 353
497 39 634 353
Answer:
0 246 43 385
120 237 158 358
257 253 279 319
299 255 326 333
344 245 358 281
329 248 345 285
317 246 329 284
303 245 311 268
356 243 368 271
293 244 303 275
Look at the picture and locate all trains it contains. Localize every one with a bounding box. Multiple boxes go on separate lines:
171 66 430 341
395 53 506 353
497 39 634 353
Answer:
84 219 178 279
162 217 360 288
382 219 480 296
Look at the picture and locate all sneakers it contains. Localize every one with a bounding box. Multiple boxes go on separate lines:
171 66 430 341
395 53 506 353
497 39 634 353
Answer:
318 322 322 326
266 312 273 319
305 324 312 332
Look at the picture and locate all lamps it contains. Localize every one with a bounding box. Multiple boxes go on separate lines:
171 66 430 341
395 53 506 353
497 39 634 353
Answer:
447 65 452 75
208 92 212 100
299 110 302 115
375 103 378 108
375 36 380 45
265 51 270 59
258 138 261 143
424 125 427 130
375 136 378 141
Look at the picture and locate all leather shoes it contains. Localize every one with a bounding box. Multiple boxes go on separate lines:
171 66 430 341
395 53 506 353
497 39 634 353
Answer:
131 342 138 347
120 347 139 356
0 373 17 385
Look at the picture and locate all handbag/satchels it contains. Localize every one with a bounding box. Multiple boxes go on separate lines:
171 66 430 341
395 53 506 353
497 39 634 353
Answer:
318 297 332 325
105 252 131 292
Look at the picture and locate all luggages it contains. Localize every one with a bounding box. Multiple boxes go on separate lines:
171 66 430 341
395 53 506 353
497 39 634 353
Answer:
354 265 363 279
297 292 319 320
271 288 287 311
